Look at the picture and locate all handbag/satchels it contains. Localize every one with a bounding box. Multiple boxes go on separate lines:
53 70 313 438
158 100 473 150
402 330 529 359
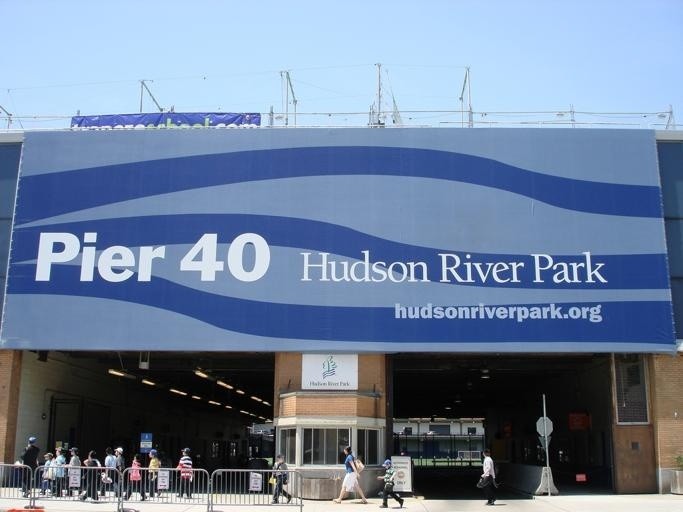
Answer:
354 459 364 473
476 478 489 488
385 482 393 493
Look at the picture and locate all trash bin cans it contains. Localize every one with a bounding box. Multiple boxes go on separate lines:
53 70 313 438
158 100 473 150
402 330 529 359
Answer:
248 459 268 493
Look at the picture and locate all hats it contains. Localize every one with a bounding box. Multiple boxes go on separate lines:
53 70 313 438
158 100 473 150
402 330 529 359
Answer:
277 454 285 458
114 447 124 455
29 437 37 444
44 447 79 457
382 459 392 466
151 449 157 456
181 448 190 453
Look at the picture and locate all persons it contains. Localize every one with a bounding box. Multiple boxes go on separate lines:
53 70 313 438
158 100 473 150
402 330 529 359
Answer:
374 459 405 508
477 448 501 506
267 451 292 504
11 435 196 502
331 443 368 504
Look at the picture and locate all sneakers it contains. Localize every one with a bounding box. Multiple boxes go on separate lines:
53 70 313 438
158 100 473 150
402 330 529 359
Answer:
116 489 192 501
488 496 496 505
333 499 341 504
400 499 403 508
379 504 388 508
272 495 292 504
21 488 106 503
360 500 367 504
